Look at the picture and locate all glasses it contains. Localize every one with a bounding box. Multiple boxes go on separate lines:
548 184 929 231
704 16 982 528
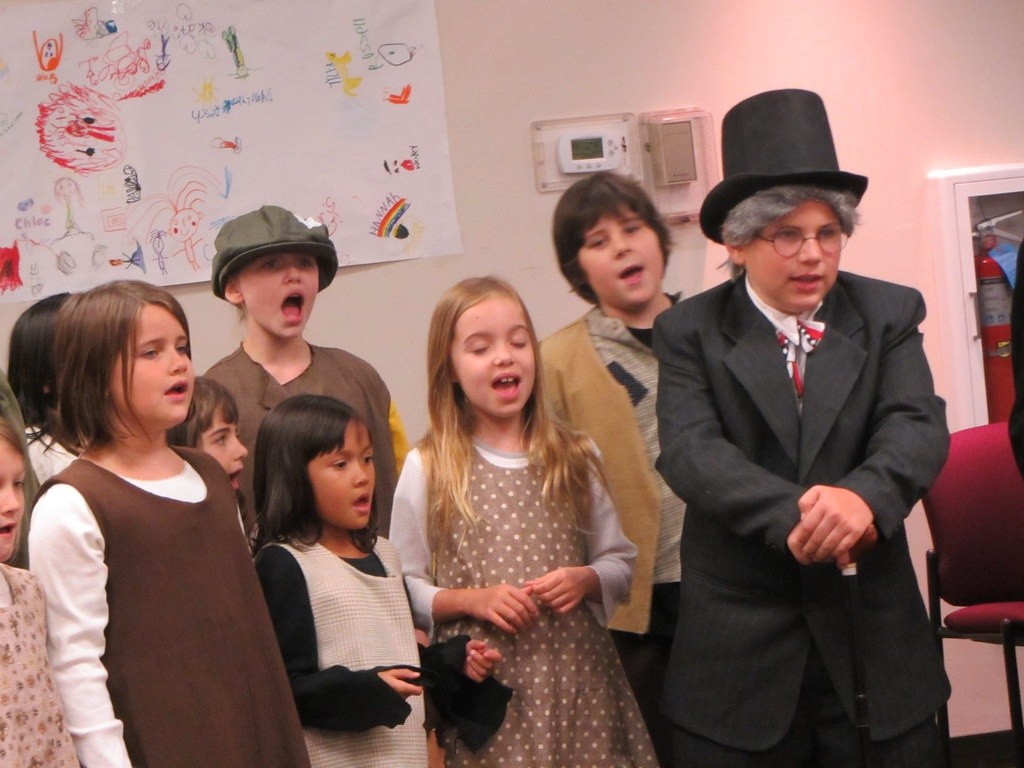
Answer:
752 222 852 258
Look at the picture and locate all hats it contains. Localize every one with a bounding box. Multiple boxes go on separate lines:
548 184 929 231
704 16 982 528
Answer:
698 88 870 248
210 206 339 308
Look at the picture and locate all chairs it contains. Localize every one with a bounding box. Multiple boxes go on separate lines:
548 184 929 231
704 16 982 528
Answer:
921 424 1023 760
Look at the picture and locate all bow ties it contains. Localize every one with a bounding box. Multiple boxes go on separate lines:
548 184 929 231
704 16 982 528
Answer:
776 317 826 402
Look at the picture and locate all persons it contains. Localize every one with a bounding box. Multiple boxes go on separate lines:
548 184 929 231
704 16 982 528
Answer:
244 394 505 767
196 206 414 540
24 280 311 768
1 421 82 768
649 89 957 768
524 174 693 768
388 277 659 767
162 379 256 547
0 293 96 492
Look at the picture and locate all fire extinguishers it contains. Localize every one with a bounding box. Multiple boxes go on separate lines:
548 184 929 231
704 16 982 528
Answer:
973 234 1019 427
